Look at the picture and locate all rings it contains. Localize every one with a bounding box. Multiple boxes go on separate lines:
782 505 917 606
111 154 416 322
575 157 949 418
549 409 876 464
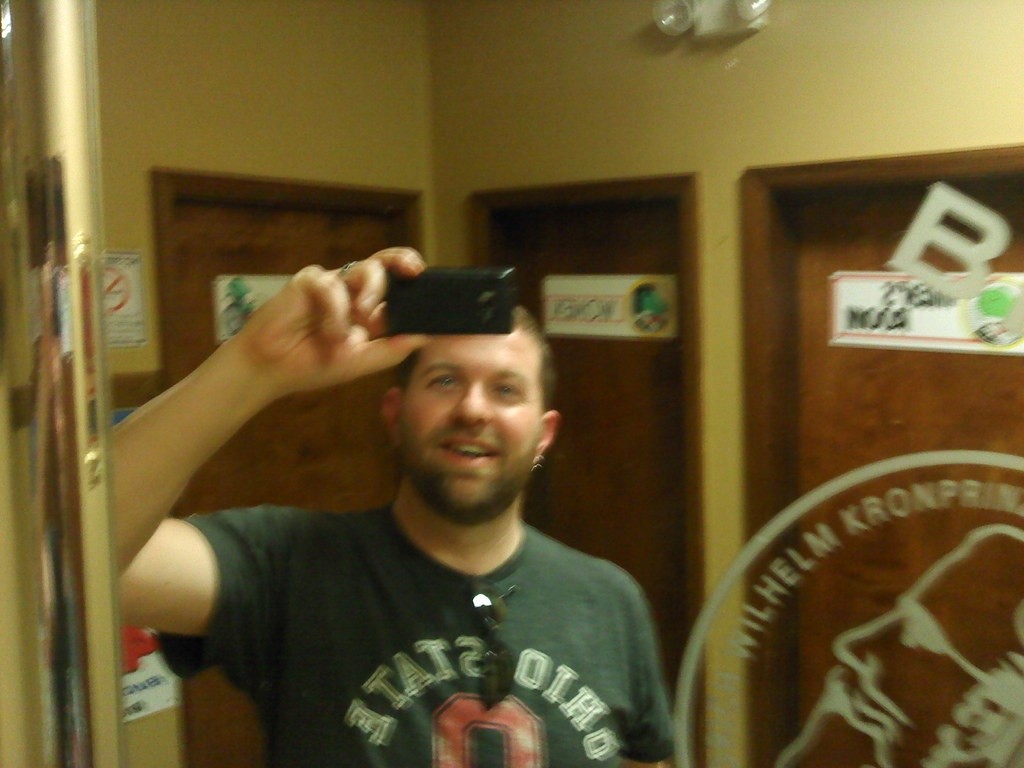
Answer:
338 260 358 277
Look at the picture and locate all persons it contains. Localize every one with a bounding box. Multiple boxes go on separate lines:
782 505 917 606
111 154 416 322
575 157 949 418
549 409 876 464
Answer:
110 247 676 768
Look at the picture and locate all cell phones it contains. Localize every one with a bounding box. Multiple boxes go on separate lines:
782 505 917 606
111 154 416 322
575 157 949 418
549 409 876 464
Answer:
386 266 515 336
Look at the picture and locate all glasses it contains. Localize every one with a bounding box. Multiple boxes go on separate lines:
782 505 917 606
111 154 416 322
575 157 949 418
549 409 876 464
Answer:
470 575 521 712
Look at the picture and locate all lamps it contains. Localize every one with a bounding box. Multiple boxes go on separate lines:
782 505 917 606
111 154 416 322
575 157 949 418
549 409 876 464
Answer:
653 0 770 41
